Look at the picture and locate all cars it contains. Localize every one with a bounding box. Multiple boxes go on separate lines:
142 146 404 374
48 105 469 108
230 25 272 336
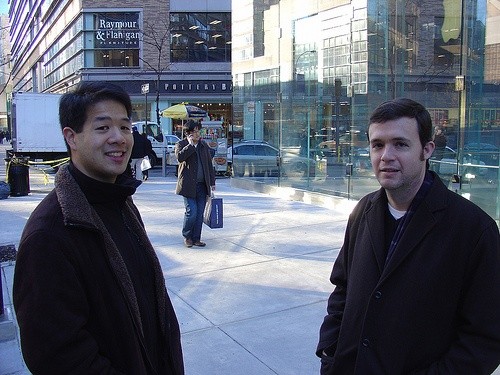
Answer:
227 139 316 177
165 134 182 158
315 127 370 148
454 143 499 157
440 147 486 176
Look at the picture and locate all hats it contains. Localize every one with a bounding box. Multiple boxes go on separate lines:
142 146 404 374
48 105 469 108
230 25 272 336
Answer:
132 127 137 131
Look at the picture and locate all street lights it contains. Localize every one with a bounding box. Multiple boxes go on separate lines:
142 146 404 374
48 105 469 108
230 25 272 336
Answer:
295 49 317 98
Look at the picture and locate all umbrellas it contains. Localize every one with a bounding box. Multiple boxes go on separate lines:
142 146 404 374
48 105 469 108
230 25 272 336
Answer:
159 102 207 139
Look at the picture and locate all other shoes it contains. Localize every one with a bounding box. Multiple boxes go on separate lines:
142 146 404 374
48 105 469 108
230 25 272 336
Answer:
144 176 147 181
192 241 206 246
184 238 192 246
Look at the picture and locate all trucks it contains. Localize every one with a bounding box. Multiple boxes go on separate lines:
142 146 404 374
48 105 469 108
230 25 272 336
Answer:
131 121 167 167
3 90 67 162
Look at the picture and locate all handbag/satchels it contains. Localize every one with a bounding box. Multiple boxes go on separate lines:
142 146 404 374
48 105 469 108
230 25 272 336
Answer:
203 191 224 228
141 156 151 171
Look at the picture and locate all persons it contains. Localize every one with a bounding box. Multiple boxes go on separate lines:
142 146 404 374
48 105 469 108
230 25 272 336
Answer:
141 132 152 180
315 97 500 375
175 119 215 247
432 126 447 173
0 131 11 144
300 128 323 160
129 126 149 181
13 80 185 375
